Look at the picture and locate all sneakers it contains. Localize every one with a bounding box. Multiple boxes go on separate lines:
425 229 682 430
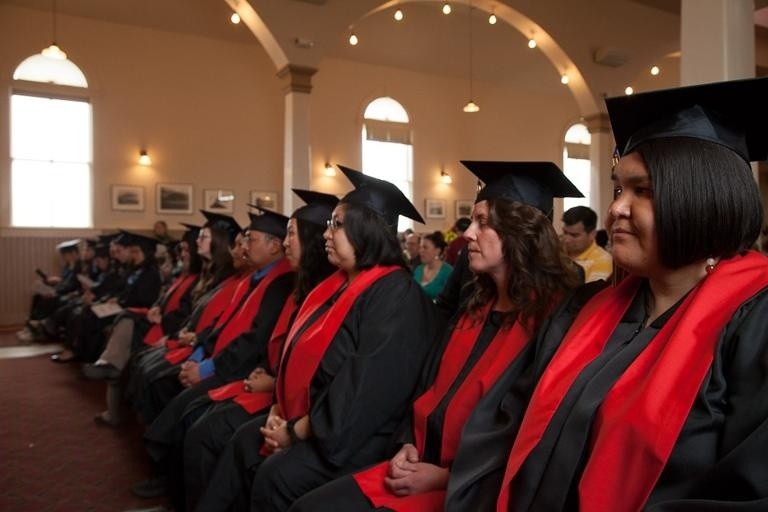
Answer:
94 412 110 423
16 317 47 345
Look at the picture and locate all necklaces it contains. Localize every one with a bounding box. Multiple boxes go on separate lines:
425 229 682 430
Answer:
705 255 715 273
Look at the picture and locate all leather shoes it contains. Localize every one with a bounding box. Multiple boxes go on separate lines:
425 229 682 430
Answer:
51 352 75 363
82 363 122 383
130 479 167 499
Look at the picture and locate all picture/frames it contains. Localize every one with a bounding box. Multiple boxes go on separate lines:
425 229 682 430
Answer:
154 182 195 215
203 188 235 214
110 184 146 211
454 199 474 220
425 198 446 220
249 189 280 215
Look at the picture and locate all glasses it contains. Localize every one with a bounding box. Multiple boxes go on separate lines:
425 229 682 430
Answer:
326 220 341 230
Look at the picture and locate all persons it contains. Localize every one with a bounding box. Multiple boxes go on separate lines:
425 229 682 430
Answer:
13 163 470 509
442 74 767 512
288 160 586 512
559 205 613 284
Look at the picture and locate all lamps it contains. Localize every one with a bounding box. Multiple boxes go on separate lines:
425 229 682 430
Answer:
324 162 337 176
441 170 452 183
138 148 150 164
463 1 479 113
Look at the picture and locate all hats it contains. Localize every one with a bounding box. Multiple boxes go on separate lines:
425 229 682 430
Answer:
245 204 289 241
337 163 425 225
55 227 156 251
177 209 243 246
603 77 764 159
290 185 339 226
459 156 585 220
